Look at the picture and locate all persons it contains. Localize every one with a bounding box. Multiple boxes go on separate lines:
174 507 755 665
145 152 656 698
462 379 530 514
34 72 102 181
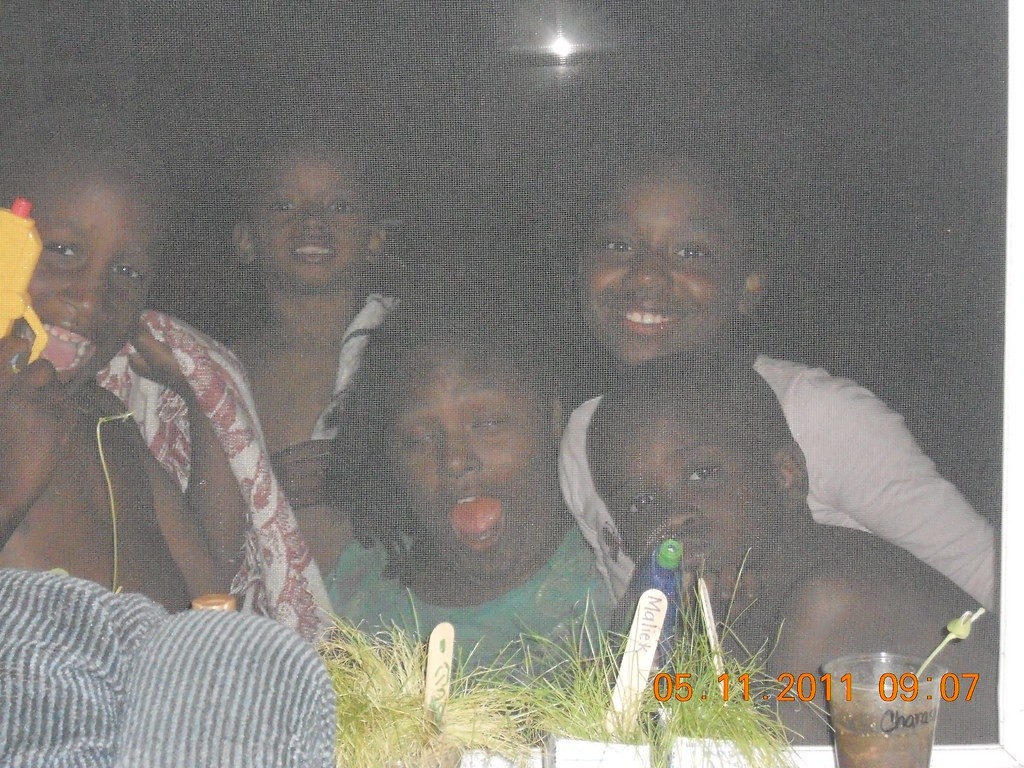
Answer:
215 133 420 580
1 99 248 616
584 350 999 745
555 135 999 622
320 283 632 697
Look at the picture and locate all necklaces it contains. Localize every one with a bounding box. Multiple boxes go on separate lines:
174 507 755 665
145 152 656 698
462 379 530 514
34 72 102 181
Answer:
260 344 330 402
